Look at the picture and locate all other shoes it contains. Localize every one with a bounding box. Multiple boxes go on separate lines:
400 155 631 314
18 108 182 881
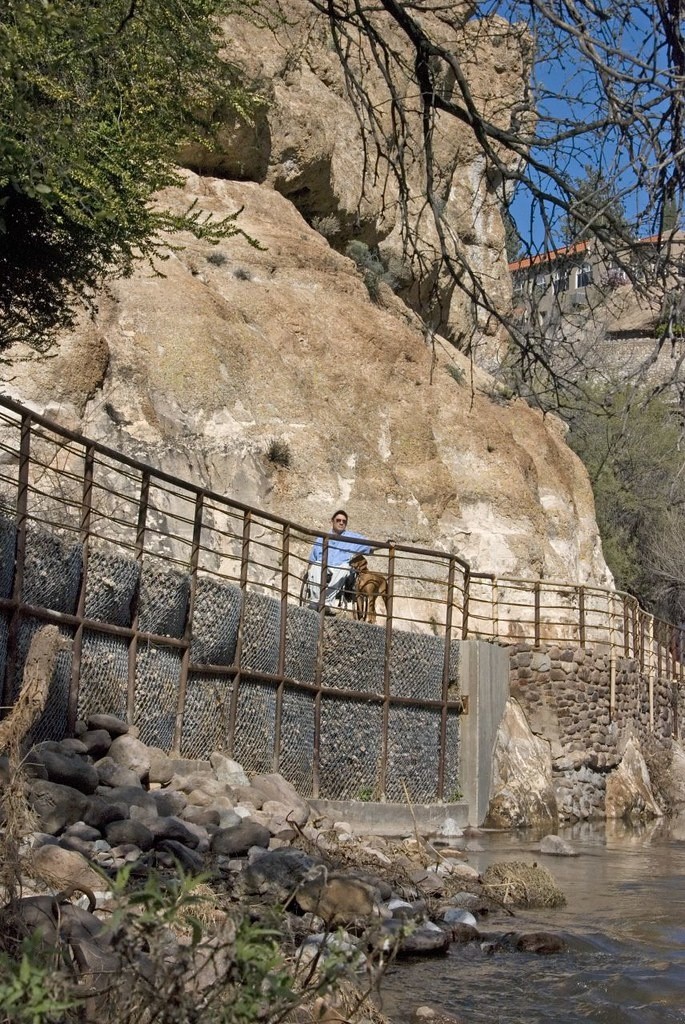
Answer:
308 604 319 612
325 606 330 614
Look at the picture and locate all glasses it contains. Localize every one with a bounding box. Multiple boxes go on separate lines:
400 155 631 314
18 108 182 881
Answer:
333 518 347 524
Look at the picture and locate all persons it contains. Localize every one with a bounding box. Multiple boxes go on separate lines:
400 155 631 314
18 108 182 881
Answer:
304 509 396 617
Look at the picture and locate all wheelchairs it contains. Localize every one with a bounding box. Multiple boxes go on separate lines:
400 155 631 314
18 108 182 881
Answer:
300 572 368 622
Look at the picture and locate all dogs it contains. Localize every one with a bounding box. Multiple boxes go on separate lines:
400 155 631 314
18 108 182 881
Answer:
349 552 389 625
